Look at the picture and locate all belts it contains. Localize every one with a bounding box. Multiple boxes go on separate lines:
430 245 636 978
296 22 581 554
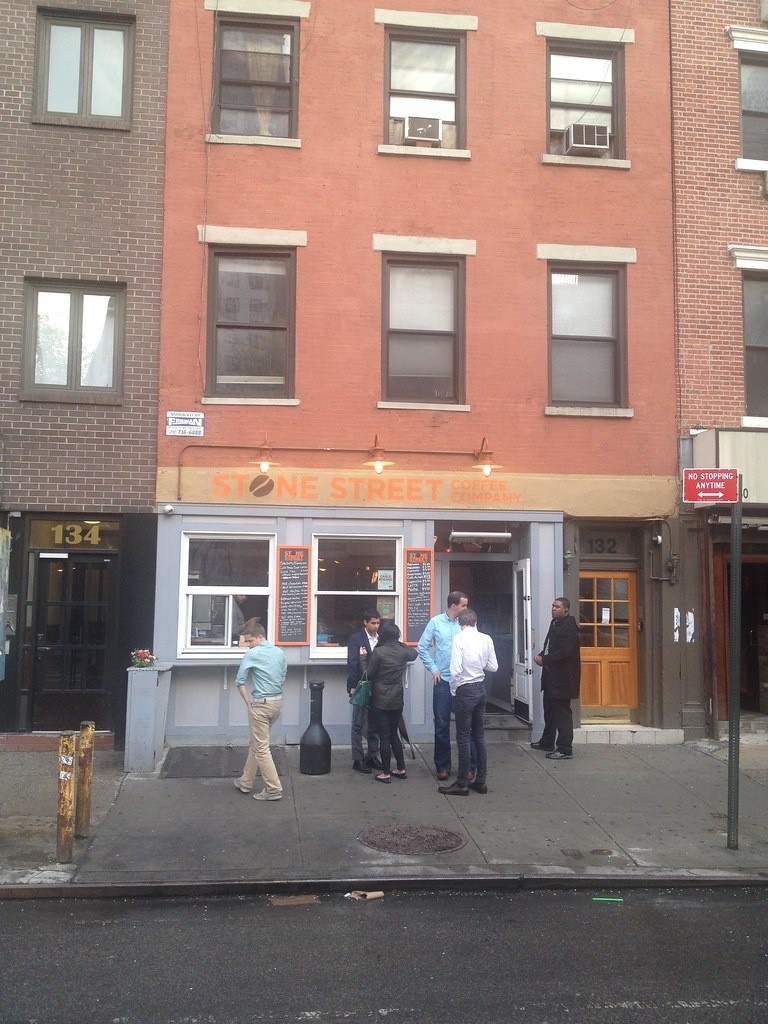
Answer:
253 695 282 701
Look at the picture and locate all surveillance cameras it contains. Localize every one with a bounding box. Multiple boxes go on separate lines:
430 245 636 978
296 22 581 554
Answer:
651 535 662 545
164 505 175 514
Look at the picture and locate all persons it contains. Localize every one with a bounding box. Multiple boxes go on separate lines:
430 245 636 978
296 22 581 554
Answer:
438 609 499 796
530 597 581 759
233 617 289 801
233 595 247 632
345 609 382 773
416 591 476 781
359 622 419 784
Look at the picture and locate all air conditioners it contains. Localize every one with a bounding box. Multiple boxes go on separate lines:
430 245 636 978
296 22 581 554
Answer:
402 116 443 146
563 122 609 157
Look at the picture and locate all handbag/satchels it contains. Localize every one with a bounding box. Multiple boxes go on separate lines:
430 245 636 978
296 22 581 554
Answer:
349 669 372 706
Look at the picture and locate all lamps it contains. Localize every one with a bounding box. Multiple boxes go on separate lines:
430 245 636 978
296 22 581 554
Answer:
247 430 281 474
361 434 396 474
471 436 504 477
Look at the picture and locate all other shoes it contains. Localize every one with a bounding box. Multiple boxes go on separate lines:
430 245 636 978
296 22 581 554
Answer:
468 772 475 781
546 751 572 759
375 774 391 783
438 770 448 780
390 769 407 778
530 742 555 751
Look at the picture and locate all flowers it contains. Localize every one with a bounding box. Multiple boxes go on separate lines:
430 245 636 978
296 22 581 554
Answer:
130 649 159 669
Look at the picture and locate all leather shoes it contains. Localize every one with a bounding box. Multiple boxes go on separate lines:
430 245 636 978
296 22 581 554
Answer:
468 782 487 794
438 783 468 796
353 762 372 773
365 757 382 769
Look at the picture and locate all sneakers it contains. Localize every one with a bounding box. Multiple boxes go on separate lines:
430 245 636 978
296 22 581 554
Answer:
234 779 250 793
253 788 282 800
